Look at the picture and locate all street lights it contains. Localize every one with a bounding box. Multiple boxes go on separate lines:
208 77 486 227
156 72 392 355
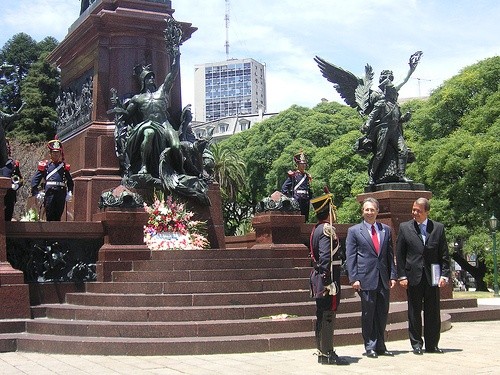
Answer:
489 212 500 300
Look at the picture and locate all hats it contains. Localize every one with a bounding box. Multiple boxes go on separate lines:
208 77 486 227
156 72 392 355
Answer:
311 186 334 213
294 147 307 164
47 134 63 152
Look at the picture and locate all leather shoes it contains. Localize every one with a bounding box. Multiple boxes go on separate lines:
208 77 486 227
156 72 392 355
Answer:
377 351 394 356
425 347 443 353
414 347 422 354
367 350 378 358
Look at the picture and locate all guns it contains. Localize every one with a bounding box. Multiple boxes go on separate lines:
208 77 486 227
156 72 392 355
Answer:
38 182 46 221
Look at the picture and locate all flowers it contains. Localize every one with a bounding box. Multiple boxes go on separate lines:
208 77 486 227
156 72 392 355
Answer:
143 187 211 251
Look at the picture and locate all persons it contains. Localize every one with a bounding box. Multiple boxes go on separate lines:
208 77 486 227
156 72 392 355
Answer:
110 42 186 178
31 140 74 221
396 197 450 355
345 197 394 358
182 110 197 143
360 50 424 187
281 154 313 224
308 194 349 365
0 159 23 221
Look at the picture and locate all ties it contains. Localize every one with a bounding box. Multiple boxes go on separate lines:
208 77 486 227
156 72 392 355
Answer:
371 225 380 254
420 224 426 246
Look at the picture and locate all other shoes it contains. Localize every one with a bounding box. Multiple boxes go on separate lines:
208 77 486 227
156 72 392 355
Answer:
318 351 349 365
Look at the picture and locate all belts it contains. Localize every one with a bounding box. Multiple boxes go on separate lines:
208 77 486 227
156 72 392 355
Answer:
46 182 65 186
296 190 308 194
333 259 342 265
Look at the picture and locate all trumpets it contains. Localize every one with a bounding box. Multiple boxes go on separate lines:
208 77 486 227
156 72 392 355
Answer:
12 175 20 184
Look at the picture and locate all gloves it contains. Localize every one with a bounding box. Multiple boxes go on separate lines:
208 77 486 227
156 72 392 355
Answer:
12 184 18 190
38 190 45 196
326 282 337 295
66 191 73 200
